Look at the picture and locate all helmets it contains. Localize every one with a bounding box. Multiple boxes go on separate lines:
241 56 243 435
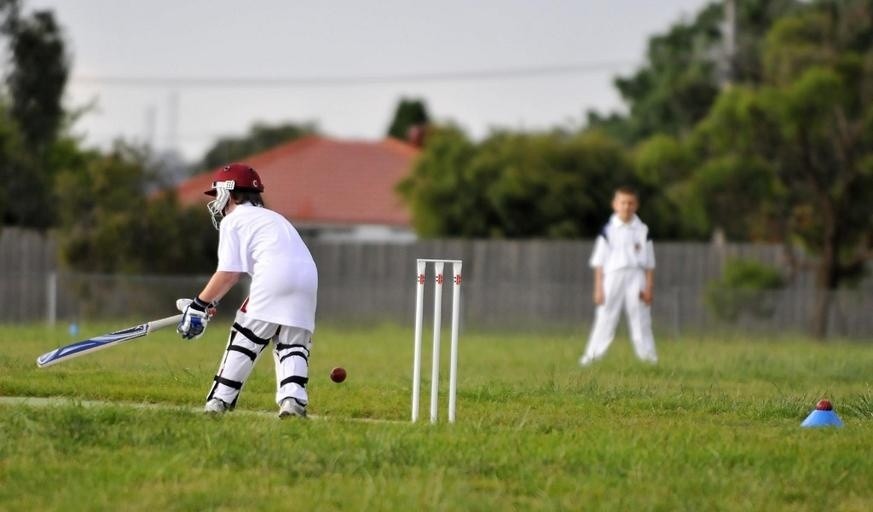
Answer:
204 163 264 197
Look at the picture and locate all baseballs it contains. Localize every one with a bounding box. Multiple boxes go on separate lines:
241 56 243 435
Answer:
329 367 346 382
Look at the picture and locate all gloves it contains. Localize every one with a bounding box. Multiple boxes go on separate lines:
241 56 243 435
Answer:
176 297 217 340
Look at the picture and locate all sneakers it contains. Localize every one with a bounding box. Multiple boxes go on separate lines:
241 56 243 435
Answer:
279 398 308 419
205 398 226 415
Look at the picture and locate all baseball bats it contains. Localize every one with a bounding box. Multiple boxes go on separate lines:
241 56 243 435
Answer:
35 306 216 369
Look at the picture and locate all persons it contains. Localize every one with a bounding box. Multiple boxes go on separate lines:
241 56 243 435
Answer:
578 185 659 366
176 163 318 420
